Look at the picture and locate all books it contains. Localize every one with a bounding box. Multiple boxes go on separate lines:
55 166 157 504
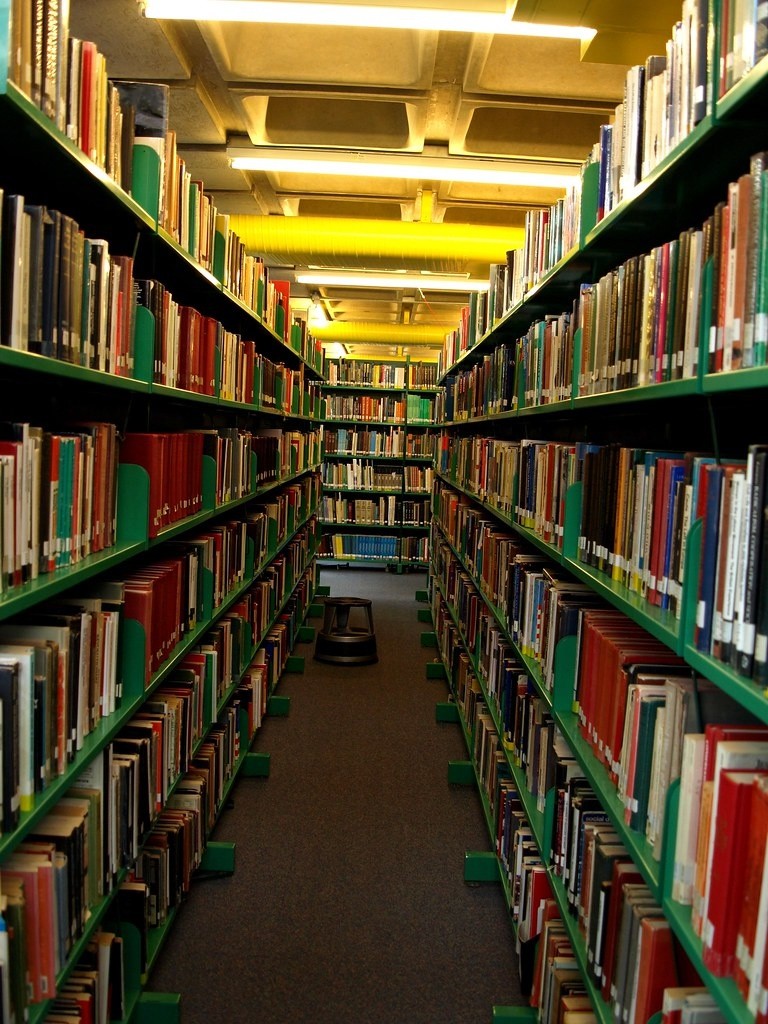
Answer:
333 361 429 574
0 0 336 1024
428 0 767 1019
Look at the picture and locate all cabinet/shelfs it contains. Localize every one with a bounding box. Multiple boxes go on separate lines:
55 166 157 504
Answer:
0 1 768 1024
